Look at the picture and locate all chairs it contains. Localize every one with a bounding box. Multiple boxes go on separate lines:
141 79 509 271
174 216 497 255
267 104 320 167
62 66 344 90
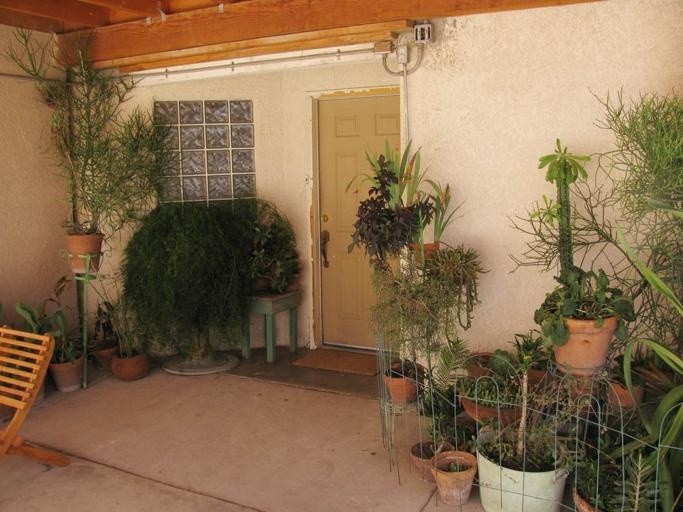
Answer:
0 324 71 468
